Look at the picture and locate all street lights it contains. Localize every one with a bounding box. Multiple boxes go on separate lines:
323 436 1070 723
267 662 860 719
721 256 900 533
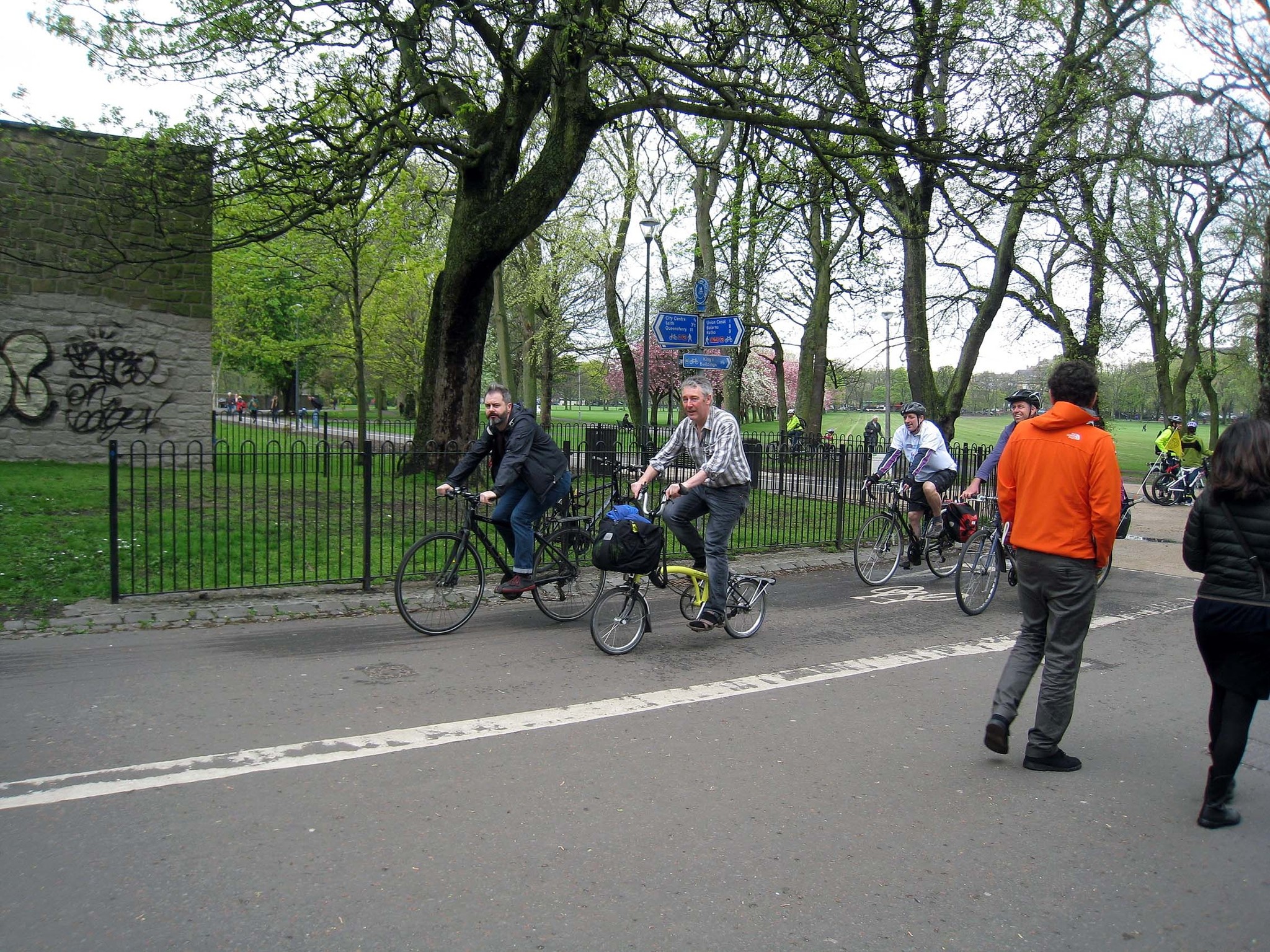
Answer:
882 311 894 449
637 219 661 474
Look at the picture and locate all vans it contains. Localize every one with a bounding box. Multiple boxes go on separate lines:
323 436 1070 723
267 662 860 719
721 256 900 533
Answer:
1227 413 1237 420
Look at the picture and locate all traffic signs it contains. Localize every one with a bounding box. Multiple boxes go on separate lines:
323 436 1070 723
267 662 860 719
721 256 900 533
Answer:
683 353 732 370
701 314 745 349
660 344 697 350
652 311 698 346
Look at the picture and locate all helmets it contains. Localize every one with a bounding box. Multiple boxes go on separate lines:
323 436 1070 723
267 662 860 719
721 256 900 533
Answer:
827 429 834 433
1167 416 1183 422
787 409 795 414
1003 389 1040 411
900 402 927 418
1187 421 1197 429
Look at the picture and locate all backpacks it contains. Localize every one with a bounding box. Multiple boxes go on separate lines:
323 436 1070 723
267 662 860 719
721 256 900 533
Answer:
793 417 806 429
1155 429 1172 456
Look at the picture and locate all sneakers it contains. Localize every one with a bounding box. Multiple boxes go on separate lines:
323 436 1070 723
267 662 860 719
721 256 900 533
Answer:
1185 501 1195 506
899 557 921 568
986 715 1009 754
1023 748 1081 772
1174 500 1184 505
497 574 536 593
926 520 943 537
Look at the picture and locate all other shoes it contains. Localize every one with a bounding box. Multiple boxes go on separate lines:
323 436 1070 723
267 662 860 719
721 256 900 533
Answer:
1158 494 1165 498
1197 804 1239 828
1221 777 1235 800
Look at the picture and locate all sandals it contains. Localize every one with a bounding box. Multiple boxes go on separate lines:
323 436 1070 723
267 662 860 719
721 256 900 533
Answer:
684 560 706 581
687 608 726 632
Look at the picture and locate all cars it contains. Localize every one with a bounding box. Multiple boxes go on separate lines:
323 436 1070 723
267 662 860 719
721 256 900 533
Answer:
1197 412 1208 418
1218 414 1223 419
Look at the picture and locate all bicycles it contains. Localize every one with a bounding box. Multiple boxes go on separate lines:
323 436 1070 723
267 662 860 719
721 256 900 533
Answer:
767 433 840 462
394 486 607 636
854 474 965 587
590 480 777 656
1142 452 1210 506
955 493 1113 616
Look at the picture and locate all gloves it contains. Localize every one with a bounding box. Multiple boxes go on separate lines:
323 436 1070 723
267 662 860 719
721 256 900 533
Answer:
1166 450 1177 455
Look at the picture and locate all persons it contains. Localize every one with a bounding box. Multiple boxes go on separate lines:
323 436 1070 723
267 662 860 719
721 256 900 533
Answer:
863 402 958 568
1183 412 1270 831
970 360 1122 771
270 396 280 423
436 383 572 593
1156 416 1183 503
865 415 885 460
235 395 247 421
1142 424 1147 432
958 389 1044 560
1170 421 1213 507
233 393 240 414
297 407 309 428
247 395 260 424
631 374 750 632
785 409 803 460
820 428 836 459
225 391 234 417
622 414 633 427
307 395 321 428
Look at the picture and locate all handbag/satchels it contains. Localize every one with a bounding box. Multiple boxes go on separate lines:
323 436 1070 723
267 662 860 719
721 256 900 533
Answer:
591 518 667 589
1116 507 1132 539
941 495 979 541
